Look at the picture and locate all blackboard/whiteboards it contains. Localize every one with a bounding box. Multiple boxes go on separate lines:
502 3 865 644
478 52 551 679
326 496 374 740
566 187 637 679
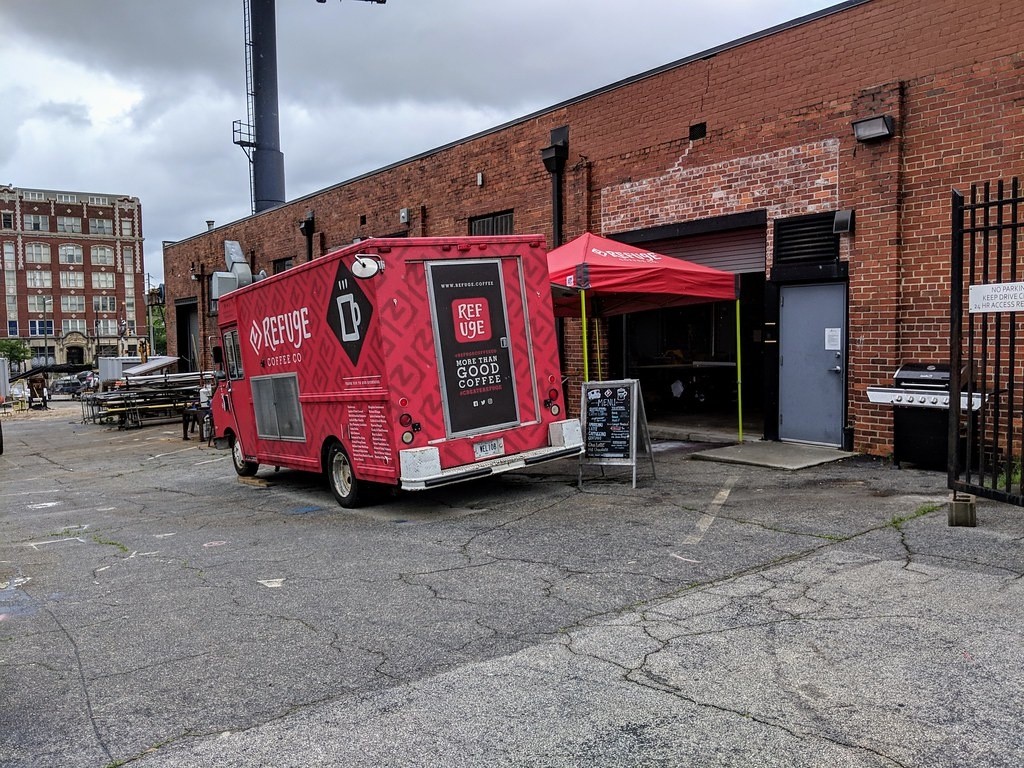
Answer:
577 379 653 465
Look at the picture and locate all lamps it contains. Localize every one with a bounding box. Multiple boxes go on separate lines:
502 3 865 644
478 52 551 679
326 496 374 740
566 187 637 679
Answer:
851 114 894 145
190 273 198 283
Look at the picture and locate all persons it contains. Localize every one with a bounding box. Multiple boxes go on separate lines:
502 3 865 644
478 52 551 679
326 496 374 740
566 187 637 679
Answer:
86 372 99 387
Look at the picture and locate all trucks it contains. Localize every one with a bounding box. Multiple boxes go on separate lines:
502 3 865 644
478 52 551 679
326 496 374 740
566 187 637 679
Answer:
196 233 588 509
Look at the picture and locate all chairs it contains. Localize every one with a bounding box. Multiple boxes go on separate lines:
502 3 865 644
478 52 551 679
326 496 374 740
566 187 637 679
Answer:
182 373 218 442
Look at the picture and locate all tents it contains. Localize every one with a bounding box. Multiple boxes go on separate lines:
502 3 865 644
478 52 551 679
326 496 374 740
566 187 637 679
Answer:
547 232 743 442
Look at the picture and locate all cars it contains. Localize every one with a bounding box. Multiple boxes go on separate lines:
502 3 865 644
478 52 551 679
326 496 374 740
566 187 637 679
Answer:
50 370 99 397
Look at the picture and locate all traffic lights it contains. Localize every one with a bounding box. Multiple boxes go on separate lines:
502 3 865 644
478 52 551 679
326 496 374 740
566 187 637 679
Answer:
126 329 132 337
147 344 152 355
